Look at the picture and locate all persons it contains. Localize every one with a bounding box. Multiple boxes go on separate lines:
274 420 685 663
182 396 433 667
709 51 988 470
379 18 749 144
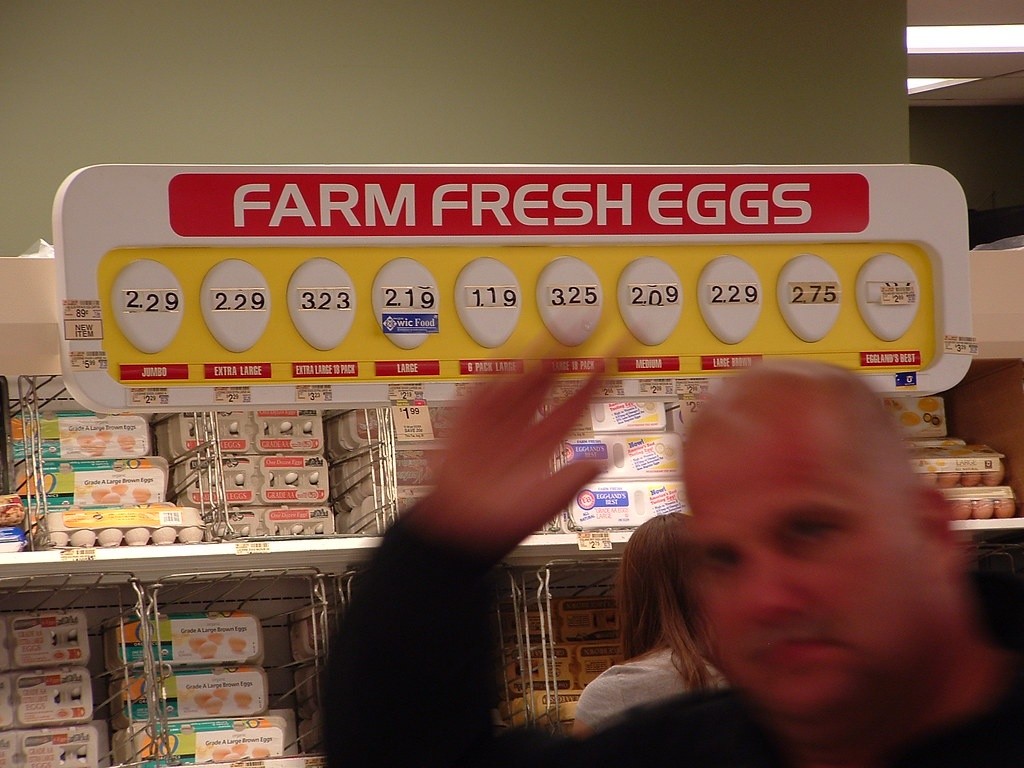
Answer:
574 512 734 738
317 326 1024 768
